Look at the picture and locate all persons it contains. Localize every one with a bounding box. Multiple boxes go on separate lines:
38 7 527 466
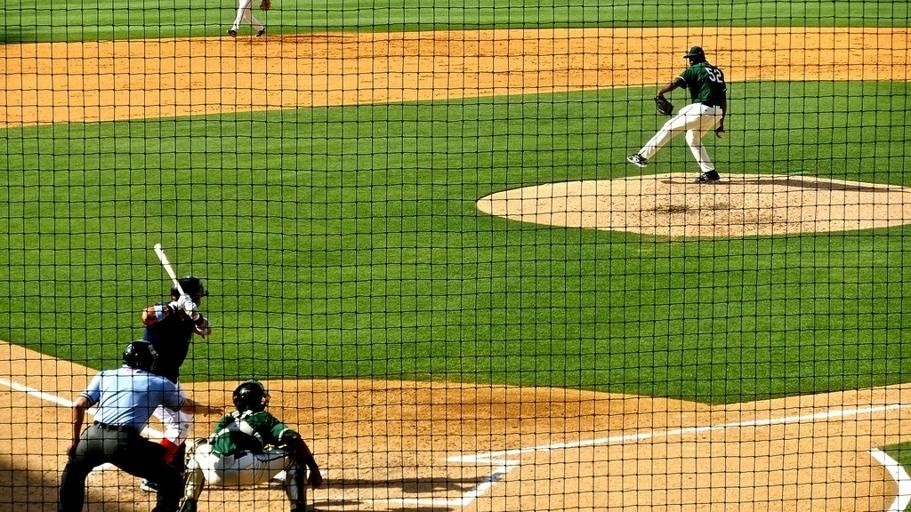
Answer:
61 340 223 512
627 45 728 182
227 0 268 36
179 381 323 511
140 276 211 493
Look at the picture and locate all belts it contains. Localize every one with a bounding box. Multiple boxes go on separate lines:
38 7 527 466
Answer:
206 449 254 462
94 421 132 431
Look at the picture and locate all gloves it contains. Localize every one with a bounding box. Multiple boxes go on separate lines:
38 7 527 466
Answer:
714 126 725 138
169 294 190 312
184 301 199 319
308 471 324 489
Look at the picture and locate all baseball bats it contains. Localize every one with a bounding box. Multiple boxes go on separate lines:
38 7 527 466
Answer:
152 244 196 319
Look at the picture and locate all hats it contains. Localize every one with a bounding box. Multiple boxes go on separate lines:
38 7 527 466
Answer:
684 46 706 60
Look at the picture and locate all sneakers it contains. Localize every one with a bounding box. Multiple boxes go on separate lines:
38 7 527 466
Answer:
179 498 198 511
256 28 266 38
627 154 649 168
140 479 158 493
696 170 720 183
226 29 237 38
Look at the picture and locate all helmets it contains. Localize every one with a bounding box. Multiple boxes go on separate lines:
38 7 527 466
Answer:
232 380 269 412
172 274 207 308
122 340 158 368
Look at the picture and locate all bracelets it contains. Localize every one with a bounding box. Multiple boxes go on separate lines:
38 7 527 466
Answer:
193 313 204 325
154 304 165 322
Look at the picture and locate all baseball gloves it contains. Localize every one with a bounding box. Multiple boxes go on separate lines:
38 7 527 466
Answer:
654 95 671 115
261 0 271 10
